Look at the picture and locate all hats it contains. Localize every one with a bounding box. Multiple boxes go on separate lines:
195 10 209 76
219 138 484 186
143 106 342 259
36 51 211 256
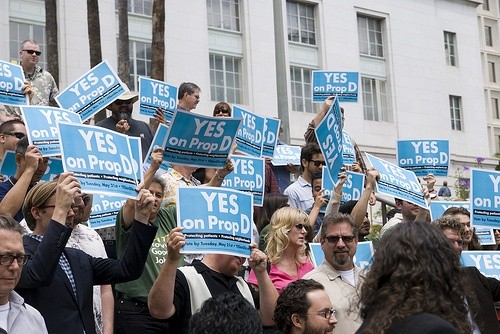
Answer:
106 83 138 110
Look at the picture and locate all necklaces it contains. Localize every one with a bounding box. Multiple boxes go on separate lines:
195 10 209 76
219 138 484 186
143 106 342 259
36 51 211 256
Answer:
8 178 15 186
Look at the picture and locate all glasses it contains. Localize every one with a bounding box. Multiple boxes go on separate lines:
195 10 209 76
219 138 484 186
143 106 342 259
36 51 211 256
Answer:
215 108 230 116
493 229 500 233
113 98 133 104
22 50 41 55
292 224 309 231
447 238 462 246
0 131 25 139
82 194 90 206
324 236 355 243
298 309 336 319
184 91 199 99
342 117 345 120
41 203 74 209
285 203 290 207
307 159 325 166
0 254 31 266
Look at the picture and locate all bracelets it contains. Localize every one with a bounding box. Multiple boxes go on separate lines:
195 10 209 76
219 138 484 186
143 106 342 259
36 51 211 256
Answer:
215 170 225 180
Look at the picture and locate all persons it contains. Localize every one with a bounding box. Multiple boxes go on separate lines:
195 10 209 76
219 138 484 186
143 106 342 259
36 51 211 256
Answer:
0 37 500 334
353 219 475 334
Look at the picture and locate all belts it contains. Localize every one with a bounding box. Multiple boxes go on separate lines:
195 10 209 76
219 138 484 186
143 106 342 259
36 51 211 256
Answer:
116 291 144 305
103 240 116 246
